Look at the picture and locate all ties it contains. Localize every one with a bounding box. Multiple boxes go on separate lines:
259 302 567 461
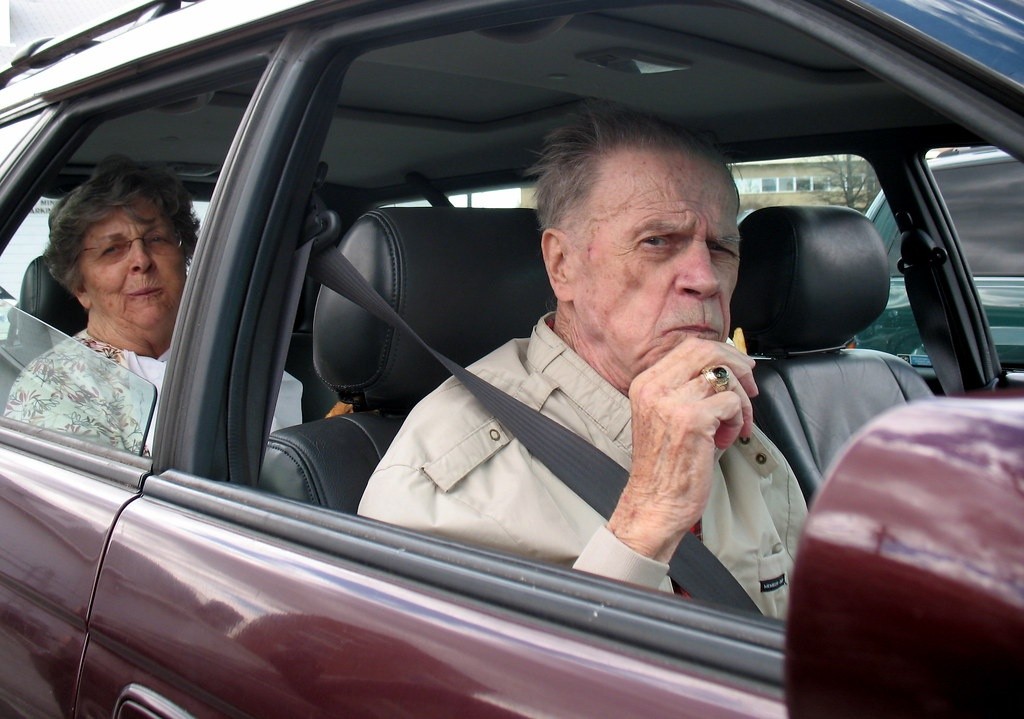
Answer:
670 517 704 599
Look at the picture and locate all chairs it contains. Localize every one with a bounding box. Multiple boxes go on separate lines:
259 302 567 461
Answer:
0 204 965 518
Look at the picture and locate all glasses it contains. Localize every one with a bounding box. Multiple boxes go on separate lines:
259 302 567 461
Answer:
76 224 185 266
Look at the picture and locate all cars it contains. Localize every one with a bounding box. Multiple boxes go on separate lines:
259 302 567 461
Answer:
0 1 1024 719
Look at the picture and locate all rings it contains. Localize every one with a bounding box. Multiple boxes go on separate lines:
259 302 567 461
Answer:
700 364 730 393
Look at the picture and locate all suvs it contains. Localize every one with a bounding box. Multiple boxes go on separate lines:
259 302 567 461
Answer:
850 148 1023 378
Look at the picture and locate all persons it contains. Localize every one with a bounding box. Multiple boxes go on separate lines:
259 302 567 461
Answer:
352 105 811 627
4 156 307 465
